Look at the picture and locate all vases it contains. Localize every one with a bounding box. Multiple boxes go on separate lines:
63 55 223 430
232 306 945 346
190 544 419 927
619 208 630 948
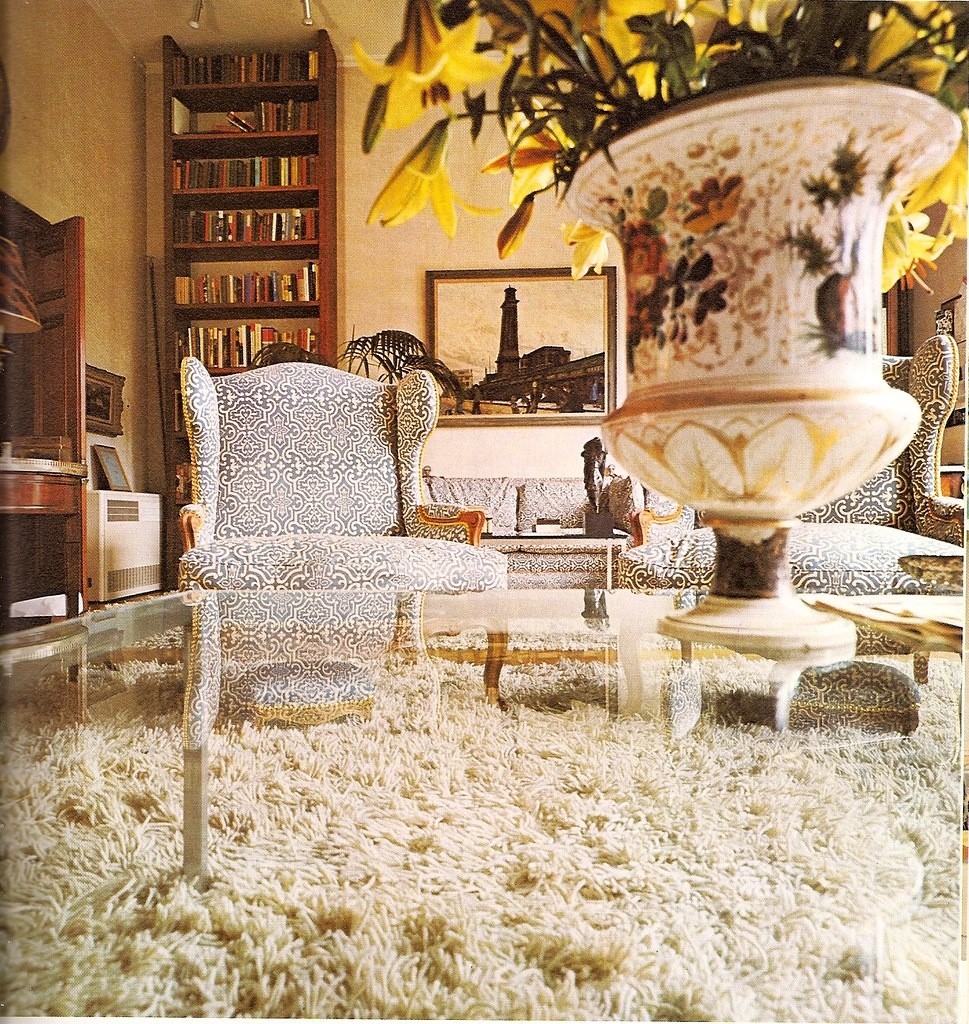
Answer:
567 66 961 658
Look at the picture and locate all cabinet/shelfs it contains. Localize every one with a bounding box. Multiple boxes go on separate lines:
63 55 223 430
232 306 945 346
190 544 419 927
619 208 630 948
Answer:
146 31 346 593
0 189 87 713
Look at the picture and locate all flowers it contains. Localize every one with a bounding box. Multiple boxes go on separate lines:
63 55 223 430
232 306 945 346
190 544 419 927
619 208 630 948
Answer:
351 0 969 296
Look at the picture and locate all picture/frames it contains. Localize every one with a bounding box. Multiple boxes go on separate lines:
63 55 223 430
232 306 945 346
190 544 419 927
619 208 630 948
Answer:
424 267 614 429
91 443 131 492
85 362 127 439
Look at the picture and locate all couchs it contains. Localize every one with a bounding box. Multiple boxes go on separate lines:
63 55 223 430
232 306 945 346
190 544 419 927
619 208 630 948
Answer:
418 471 697 591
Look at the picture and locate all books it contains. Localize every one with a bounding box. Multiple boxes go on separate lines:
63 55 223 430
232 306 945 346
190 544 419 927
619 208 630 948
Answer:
169 46 319 510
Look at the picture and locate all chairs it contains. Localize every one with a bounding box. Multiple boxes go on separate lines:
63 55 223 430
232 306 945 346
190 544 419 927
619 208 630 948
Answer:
177 358 509 714
580 326 964 684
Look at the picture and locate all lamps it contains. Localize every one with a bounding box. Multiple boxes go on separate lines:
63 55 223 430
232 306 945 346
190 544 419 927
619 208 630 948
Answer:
0 237 43 355
301 0 316 26
189 0 203 28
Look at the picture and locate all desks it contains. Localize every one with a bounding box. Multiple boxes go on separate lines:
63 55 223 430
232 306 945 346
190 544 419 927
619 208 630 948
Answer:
0 589 966 1023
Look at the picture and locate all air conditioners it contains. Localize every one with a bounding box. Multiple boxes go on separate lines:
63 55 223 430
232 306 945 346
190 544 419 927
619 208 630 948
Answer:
87 490 162 604
